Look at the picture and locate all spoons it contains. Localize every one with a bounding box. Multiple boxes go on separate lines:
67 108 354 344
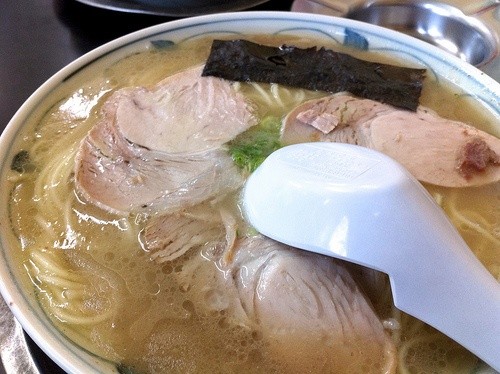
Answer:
238 145 500 372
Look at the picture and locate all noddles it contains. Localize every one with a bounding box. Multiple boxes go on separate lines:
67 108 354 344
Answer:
15 35 499 374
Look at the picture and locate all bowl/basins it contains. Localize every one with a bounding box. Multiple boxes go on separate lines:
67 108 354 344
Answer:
344 1 496 74
0 7 500 373
